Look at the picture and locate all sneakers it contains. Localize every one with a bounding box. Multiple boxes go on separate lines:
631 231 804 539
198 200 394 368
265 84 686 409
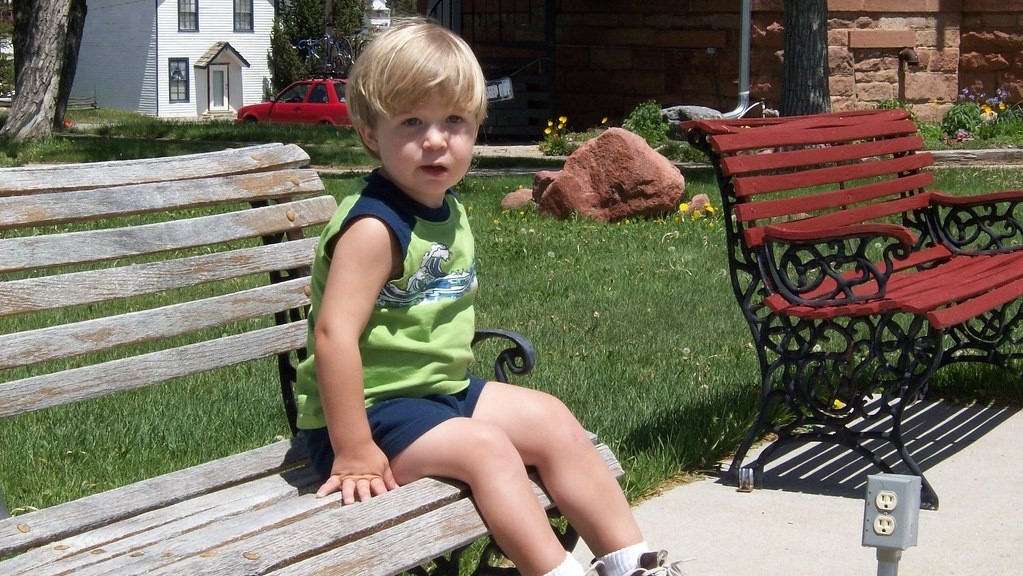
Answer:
585 548 697 576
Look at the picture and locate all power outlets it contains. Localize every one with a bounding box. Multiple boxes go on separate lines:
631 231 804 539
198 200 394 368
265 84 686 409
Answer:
859 471 923 552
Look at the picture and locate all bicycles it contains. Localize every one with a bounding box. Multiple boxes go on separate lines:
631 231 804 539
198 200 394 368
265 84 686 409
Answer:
296 22 372 78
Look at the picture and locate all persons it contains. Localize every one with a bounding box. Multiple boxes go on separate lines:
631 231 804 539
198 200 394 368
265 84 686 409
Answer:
296 22 688 576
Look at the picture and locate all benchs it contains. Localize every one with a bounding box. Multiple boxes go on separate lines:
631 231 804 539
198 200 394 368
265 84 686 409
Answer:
663 105 1022 510
0 137 626 576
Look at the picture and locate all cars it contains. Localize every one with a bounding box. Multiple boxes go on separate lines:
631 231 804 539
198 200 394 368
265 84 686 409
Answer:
233 76 354 128
